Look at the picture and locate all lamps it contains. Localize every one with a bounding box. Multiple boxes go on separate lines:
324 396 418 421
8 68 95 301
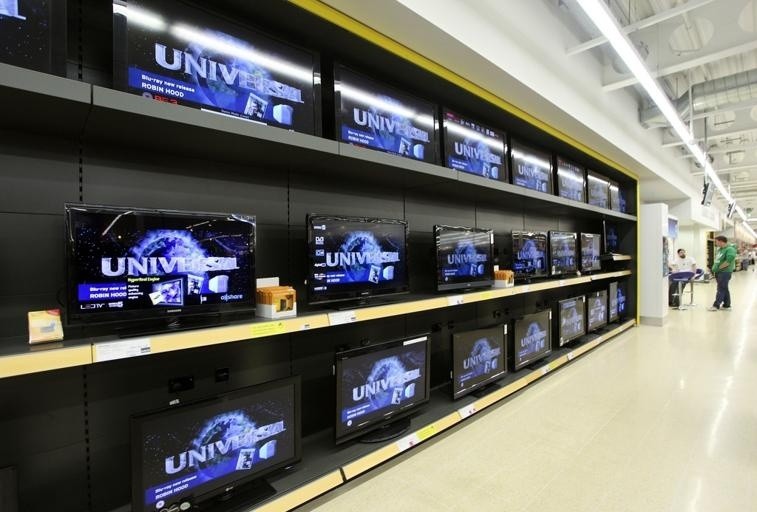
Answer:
726 152 736 220
699 116 715 205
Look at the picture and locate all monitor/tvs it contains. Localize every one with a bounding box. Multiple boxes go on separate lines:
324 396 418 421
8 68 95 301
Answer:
434 226 494 293
442 105 511 184
131 375 302 512
510 133 554 194
727 202 736 218
64 202 256 339
304 213 411 311
450 320 508 401
511 307 552 371
111 0 323 139
701 183 715 206
510 228 549 284
333 60 441 167
0 0 68 77
555 154 628 348
334 332 430 446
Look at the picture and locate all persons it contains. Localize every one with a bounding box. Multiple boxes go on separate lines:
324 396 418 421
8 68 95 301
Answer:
669 249 696 309
707 236 736 311
740 247 749 271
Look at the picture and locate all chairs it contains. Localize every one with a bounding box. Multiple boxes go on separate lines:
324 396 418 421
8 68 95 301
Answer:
684 268 704 307
668 271 695 310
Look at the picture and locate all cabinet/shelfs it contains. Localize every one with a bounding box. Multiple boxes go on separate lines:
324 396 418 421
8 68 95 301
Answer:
0 1 643 510
